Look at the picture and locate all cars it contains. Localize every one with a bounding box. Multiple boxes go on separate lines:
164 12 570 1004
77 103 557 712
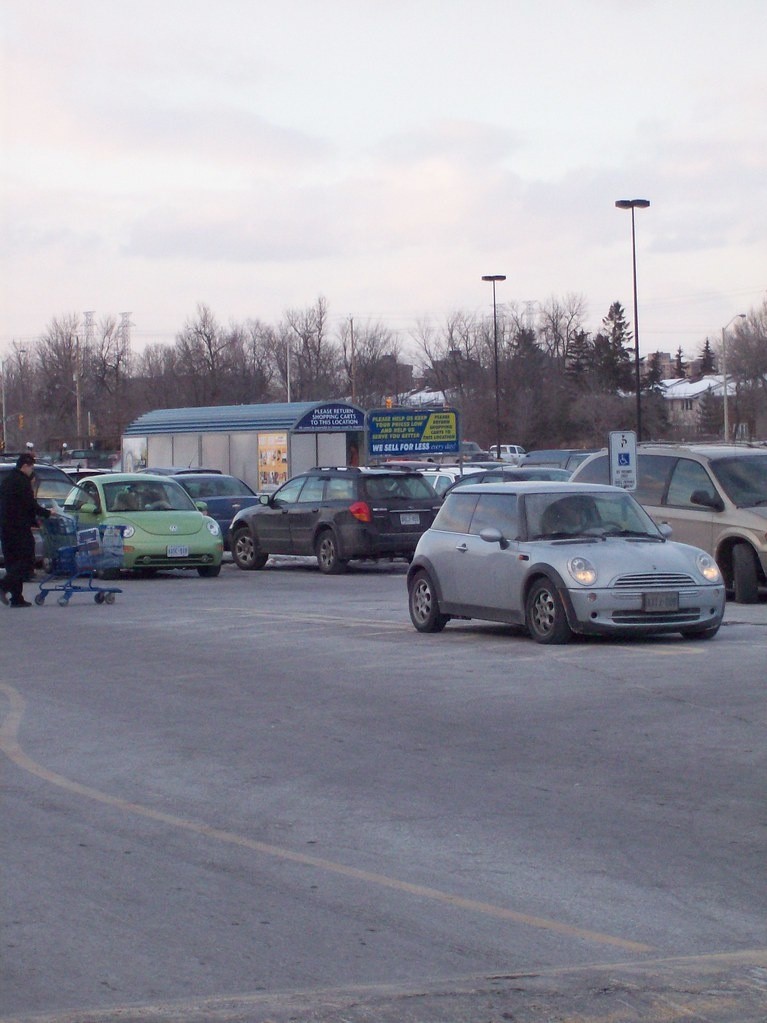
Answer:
0 461 77 570
60 467 223 496
388 448 596 500
163 473 262 552
34 473 223 576
405 482 726 644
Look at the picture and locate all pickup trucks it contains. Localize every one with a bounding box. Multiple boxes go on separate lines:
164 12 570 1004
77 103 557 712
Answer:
63 450 106 468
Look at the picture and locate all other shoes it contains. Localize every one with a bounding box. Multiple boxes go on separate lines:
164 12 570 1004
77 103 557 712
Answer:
0 590 9 605
9 598 32 608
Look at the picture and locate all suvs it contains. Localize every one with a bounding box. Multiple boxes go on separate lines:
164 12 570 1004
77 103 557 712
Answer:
489 445 527 463
228 465 445 576
568 444 767 603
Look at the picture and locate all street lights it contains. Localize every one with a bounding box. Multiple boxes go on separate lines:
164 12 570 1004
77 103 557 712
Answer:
616 199 650 444
482 275 508 451
1 350 27 453
56 384 91 435
721 314 747 444
287 328 319 403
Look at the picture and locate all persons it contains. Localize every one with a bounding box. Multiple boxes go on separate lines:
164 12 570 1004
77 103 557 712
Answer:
0 453 56 608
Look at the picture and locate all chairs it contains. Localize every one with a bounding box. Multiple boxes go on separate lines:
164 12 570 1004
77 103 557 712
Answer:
112 492 139 510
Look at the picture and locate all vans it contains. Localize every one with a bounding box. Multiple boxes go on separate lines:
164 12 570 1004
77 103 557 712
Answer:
419 442 484 465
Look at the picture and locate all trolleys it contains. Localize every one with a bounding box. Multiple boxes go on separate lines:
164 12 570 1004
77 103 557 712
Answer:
34 512 125 605
